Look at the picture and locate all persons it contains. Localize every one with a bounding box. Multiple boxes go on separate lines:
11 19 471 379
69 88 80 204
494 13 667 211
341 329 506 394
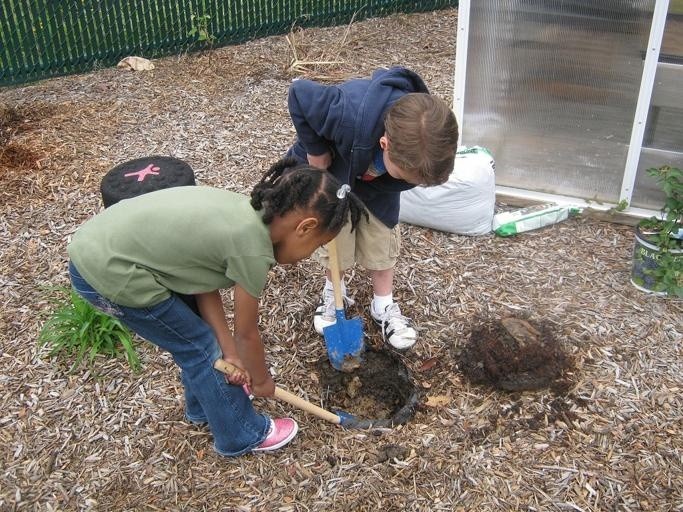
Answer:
283 64 457 352
66 163 355 455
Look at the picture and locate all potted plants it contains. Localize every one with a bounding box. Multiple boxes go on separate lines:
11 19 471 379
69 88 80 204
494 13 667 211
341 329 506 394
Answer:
630 161 683 300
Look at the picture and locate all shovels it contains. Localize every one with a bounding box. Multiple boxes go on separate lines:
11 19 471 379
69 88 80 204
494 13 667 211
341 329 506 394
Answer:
323 240 364 371
214 359 362 428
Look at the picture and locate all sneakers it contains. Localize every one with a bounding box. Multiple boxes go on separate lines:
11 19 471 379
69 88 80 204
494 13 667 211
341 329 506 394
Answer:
314 281 354 338
369 299 418 350
250 418 299 452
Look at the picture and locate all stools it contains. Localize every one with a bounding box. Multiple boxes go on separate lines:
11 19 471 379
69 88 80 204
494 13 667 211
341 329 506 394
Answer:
99 155 196 213
